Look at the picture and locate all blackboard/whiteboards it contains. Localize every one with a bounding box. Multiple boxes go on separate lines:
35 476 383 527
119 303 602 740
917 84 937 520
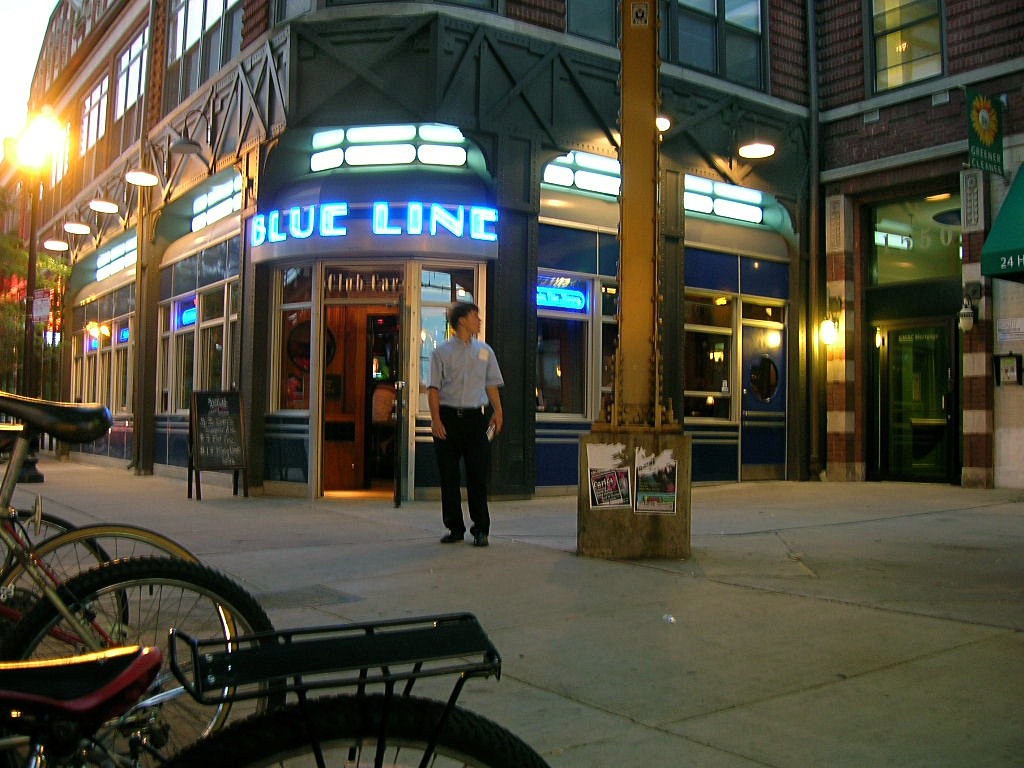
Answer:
187 390 248 472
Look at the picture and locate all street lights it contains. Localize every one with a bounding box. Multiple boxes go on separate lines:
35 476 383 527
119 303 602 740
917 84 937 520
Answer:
9 105 64 398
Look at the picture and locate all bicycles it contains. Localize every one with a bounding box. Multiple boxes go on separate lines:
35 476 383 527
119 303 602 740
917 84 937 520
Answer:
0 390 552 767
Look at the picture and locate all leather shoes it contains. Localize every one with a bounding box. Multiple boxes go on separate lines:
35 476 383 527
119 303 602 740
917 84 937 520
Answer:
441 531 465 543
474 532 489 547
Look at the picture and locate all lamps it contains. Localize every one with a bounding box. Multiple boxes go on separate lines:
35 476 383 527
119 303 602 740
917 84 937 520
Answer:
63 198 98 235
734 109 775 159
124 142 167 186
959 292 974 332
169 108 209 154
43 228 69 251
88 173 127 214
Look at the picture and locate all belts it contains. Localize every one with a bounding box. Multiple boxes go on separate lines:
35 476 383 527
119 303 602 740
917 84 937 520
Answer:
438 406 482 417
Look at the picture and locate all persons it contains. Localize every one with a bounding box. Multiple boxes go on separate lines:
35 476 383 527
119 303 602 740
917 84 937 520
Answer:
426 302 505 547
